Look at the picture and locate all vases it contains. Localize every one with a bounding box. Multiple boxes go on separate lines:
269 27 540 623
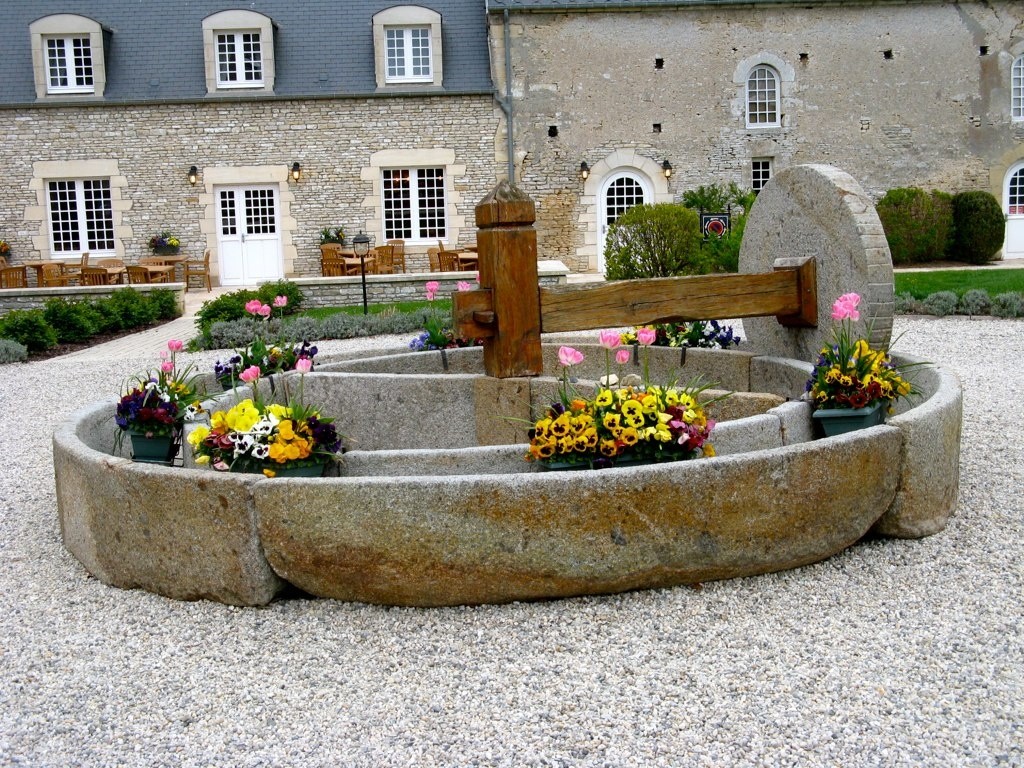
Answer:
221 381 245 391
813 402 887 437
535 452 670 470
228 460 325 477
156 247 179 255
127 430 175 461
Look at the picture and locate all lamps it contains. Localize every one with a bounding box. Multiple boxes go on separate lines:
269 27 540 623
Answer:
581 161 589 181
292 162 300 182
188 166 197 187
662 159 672 180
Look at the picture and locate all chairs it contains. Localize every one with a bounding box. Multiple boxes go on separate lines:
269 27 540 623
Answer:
427 240 477 272
0 249 212 293
320 240 406 277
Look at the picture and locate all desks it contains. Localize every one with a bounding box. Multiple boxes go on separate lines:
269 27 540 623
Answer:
123 266 175 278
22 260 64 287
463 244 477 249
77 267 124 284
138 256 189 282
324 257 375 267
338 250 354 257
454 253 478 260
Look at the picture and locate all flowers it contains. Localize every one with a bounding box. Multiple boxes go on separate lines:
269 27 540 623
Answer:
0 241 11 254
105 275 937 479
320 225 345 245
152 230 179 255
